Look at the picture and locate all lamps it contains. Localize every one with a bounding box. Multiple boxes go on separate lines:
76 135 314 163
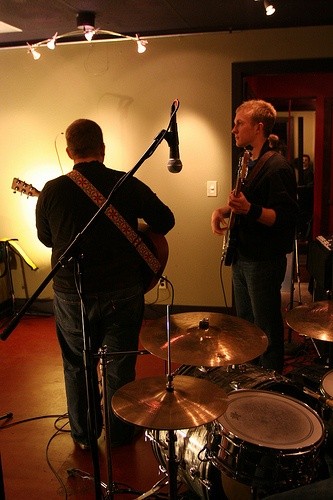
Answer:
27 24 151 60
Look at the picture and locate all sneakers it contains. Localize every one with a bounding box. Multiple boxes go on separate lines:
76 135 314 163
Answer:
69 434 98 450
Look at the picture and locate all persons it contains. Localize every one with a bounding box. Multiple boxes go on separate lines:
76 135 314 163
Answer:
36 119 176 449
302 155 313 236
212 100 298 373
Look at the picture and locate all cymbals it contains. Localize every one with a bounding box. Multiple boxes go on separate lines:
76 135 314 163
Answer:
110 375 228 430
284 300 333 341
140 312 269 365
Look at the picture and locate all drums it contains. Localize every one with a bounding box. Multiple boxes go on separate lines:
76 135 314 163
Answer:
205 388 324 493
143 359 293 500
318 368 333 399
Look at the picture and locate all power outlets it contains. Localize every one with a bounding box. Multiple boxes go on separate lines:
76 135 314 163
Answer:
159 276 166 289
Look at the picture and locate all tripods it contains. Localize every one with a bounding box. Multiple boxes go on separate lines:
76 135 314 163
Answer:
66 348 168 500
0 243 54 329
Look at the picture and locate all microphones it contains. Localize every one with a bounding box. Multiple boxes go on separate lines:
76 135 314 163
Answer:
168 104 182 173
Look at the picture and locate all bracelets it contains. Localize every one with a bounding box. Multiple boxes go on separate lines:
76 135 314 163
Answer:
248 203 262 218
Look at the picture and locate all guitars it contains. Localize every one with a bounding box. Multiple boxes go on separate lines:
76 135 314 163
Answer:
11 177 169 294
220 150 252 267
